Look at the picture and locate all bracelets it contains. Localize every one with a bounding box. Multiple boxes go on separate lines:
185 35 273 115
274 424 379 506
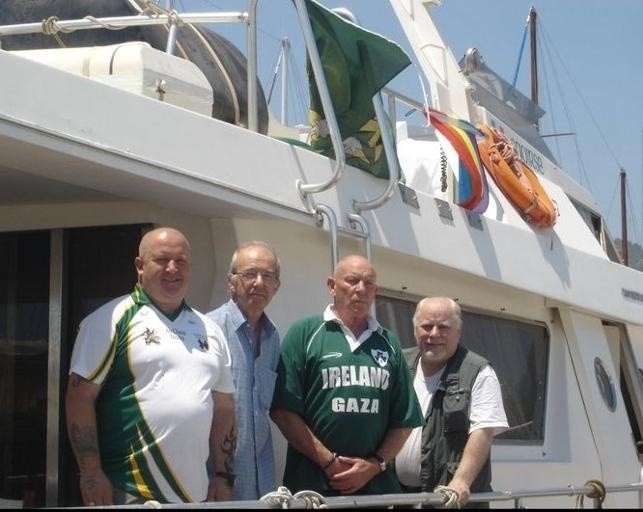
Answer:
321 451 338 471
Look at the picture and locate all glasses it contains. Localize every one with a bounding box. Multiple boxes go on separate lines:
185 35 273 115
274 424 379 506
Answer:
233 270 274 280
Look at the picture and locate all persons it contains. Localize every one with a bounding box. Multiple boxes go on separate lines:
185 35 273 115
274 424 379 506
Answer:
65 227 237 506
270 256 426 497
203 241 280 502
393 296 510 510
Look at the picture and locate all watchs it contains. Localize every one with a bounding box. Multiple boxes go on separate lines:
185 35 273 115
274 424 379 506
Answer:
215 470 237 488
373 453 386 472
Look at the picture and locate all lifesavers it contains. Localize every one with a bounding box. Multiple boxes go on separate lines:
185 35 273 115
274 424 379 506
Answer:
475 122 558 226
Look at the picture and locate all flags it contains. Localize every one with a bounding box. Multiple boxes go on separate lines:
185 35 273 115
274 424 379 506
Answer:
424 107 489 215
271 0 411 182
